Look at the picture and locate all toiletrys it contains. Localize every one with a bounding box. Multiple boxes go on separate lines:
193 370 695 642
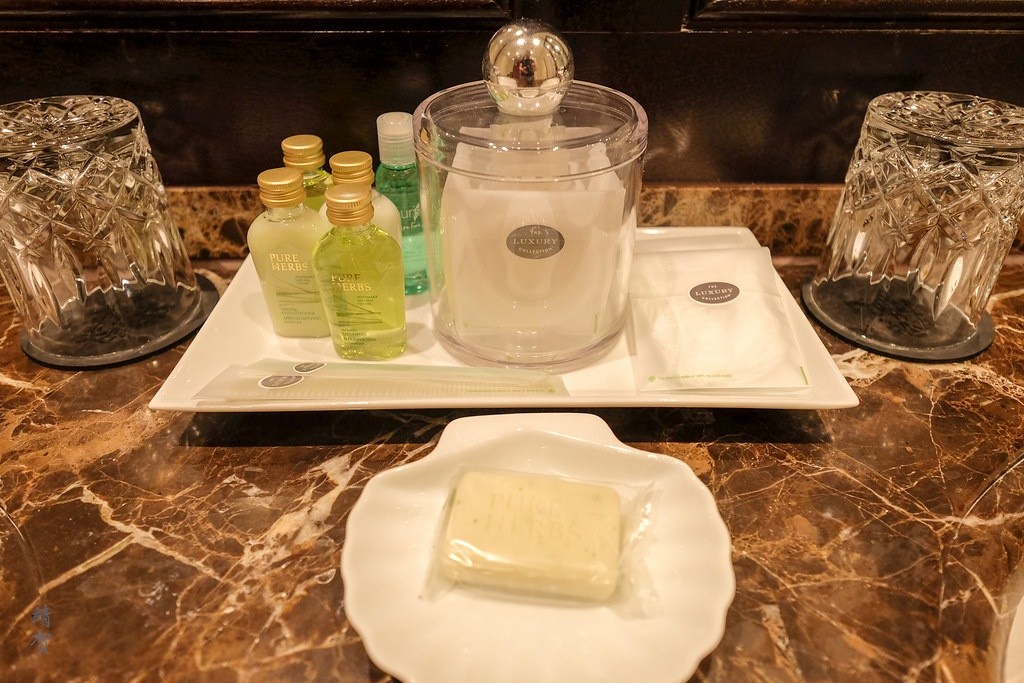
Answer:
247 111 444 363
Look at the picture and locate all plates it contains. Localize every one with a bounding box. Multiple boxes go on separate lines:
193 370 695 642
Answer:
341 411 737 683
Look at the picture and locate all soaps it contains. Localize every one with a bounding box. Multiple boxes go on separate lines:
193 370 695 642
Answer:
438 465 625 605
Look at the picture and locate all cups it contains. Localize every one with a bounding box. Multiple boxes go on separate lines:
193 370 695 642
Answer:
0 94 203 360
412 18 650 365
810 91 1024 352
